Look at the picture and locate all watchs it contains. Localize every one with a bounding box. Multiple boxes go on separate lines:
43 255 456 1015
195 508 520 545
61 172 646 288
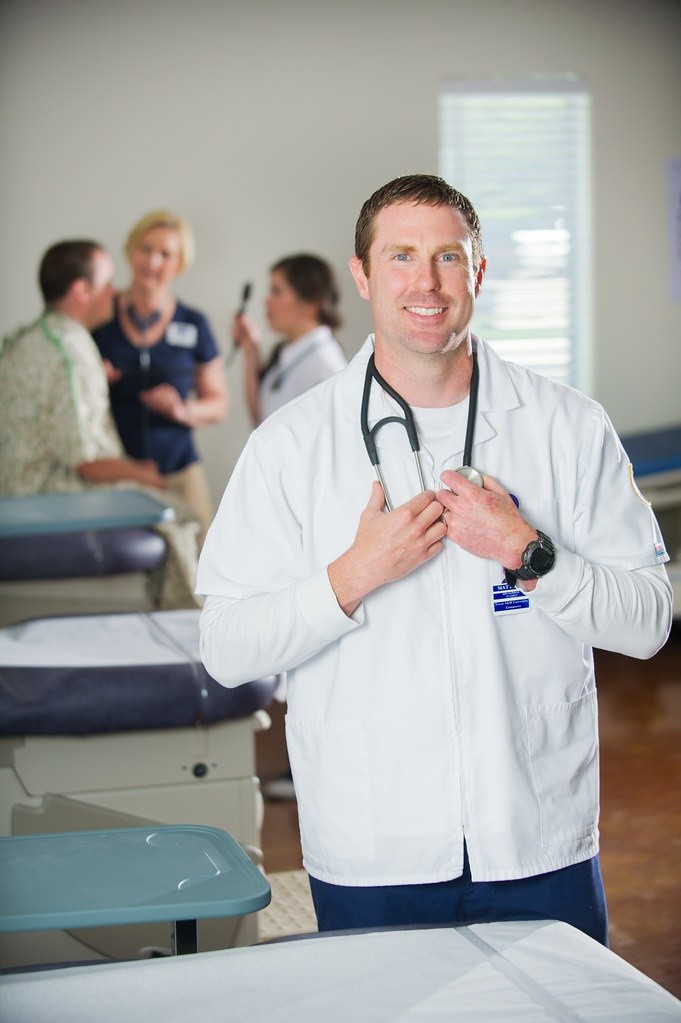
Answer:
504 528 554 590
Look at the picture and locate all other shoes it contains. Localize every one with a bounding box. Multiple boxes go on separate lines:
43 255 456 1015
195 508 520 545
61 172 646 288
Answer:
264 771 295 799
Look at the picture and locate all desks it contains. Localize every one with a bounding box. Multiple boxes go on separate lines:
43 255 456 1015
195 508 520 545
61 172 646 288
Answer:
0 486 175 613
0 824 275 977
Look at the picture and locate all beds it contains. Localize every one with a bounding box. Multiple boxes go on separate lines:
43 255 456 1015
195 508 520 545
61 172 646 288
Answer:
617 425 681 507
0 611 279 868
0 915 681 1023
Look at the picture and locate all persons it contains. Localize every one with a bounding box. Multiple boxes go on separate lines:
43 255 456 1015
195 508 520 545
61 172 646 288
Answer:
92 212 227 552
194 174 671 951
0 240 170 504
234 252 346 428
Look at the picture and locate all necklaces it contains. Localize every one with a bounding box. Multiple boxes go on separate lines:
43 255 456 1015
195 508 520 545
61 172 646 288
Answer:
122 291 162 371
